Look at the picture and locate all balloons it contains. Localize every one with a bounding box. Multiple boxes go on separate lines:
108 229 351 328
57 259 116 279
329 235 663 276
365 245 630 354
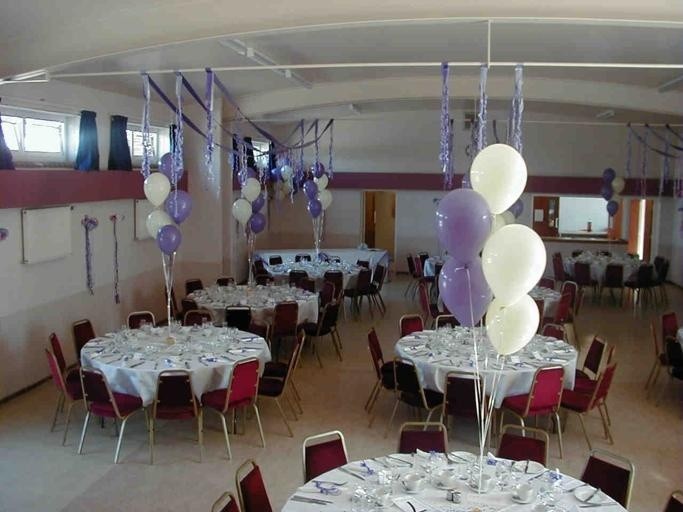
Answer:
141 152 192 260
434 186 494 270
506 198 523 220
484 290 543 356
600 167 626 220
302 162 333 223
461 171 473 189
469 144 527 215
231 165 267 234
270 153 300 204
480 221 547 311
437 253 495 331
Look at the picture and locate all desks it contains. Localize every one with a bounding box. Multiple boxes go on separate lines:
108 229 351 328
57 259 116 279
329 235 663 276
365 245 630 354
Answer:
254 247 388 285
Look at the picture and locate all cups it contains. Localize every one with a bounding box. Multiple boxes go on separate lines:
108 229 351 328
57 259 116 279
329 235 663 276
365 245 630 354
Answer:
193 279 312 307
85 317 264 371
270 255 357 274
335 451 564 511
401 323 573 369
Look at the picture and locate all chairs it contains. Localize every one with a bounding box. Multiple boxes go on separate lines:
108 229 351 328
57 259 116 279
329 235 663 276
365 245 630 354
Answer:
660 486 682 512
494 425 551 466
414 274 585 350
580 447 634 509
551 247 671 309
232 457 275 512
403 249 485 301
163 275 345 370
393 421 449 455
364 314 618 449
300 428 350 485
43 310 306 468
645 312 682 421
210 490 240 512
252 253 388 321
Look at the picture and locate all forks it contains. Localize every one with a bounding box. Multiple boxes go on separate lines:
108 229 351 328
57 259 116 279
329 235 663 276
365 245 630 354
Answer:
312 479 347 486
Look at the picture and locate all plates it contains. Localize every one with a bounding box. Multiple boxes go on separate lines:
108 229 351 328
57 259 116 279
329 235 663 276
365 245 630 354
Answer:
573 486 602 503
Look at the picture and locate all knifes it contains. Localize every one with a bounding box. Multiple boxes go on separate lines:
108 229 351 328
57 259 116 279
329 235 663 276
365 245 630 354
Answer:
566 481 590 493
290 494 336 506
583 486 601 503
578 501 616 508
